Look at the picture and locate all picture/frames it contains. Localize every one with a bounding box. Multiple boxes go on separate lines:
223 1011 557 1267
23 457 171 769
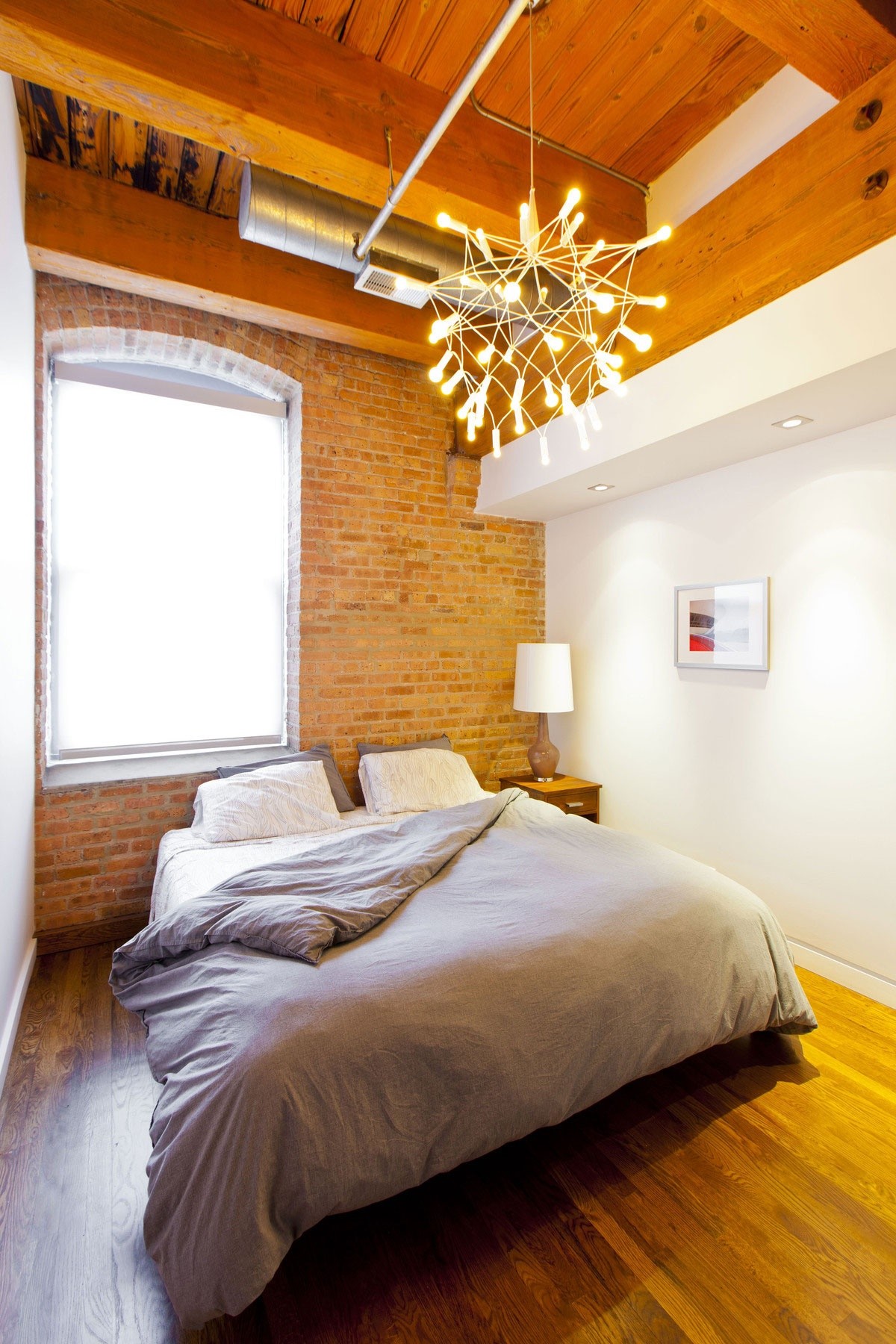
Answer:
674 576 771 671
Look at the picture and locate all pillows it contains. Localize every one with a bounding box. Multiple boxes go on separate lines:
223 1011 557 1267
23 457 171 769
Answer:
190 760 345 845
216 744 357 813
356 734 453 756
358 748 487 816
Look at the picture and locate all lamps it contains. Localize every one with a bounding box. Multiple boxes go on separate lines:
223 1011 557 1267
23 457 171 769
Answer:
514 643 575 782
393 0 672 468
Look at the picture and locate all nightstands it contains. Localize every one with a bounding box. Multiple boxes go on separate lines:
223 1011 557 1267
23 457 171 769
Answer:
499 774 603 824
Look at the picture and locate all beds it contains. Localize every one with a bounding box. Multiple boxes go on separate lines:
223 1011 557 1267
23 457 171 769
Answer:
109 790 820 1332
